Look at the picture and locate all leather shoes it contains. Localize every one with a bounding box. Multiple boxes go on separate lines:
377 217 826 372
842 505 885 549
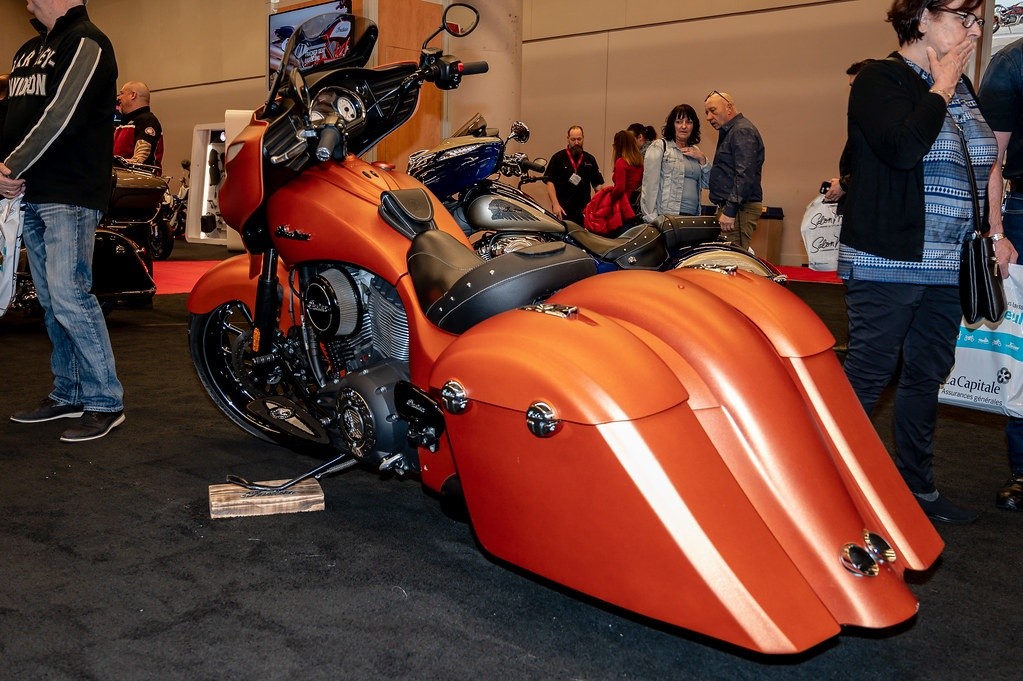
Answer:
59 409 125 441
996 474 1023 510
9 396 82 421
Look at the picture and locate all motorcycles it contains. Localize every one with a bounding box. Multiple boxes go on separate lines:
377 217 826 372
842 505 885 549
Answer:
8 154 193 313
183 2 947 657
407 111 789 287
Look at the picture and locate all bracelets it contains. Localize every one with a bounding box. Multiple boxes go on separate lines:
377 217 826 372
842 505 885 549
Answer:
929 90 952 99
990 233 1005 242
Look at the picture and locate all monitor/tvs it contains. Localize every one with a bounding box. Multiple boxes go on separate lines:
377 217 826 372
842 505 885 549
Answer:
269 0 352 91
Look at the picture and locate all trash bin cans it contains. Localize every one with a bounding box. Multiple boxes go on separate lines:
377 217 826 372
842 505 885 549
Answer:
749 206 784 266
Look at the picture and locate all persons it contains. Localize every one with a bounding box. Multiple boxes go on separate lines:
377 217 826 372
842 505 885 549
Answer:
705 89 768 250
611 130 644 197
825 58 876 352
640 104 712 223
626 123 657 157
837 0 999 524
978 36 1023 511
114 80 164 309
542 125 606 228
0 0 125 441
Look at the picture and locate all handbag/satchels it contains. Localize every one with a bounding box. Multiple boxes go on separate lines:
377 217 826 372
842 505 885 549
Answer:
935 262 1023 419
802 194 843 271
961 232 1004 325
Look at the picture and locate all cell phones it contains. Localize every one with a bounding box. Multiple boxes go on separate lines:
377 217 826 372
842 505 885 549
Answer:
820 181 831 195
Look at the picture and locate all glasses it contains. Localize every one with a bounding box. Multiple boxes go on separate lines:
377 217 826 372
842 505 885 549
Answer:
704 90 730 103
929 8 985 29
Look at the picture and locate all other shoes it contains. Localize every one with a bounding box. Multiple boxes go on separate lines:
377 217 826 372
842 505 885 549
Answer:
913 491 982 523
